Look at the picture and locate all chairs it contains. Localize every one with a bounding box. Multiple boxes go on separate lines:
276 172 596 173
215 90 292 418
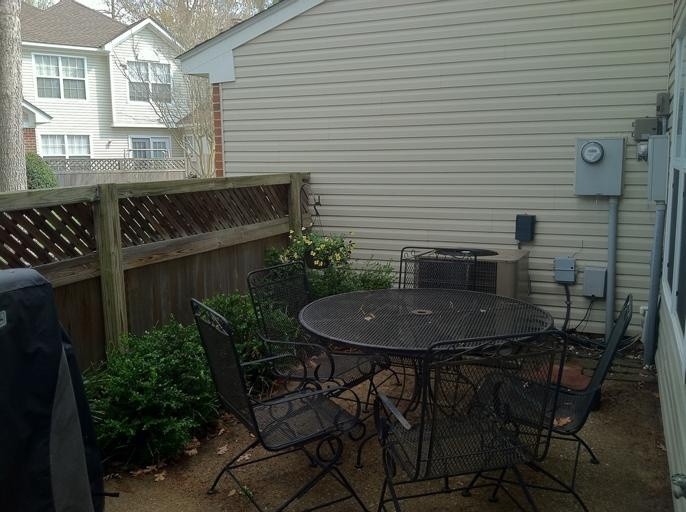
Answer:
188 297 370 511
362 245 483 426
372 329 568 512
245 262 394 465
462 292 635 512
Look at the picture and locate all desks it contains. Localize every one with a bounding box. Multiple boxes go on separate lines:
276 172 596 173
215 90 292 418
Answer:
299 287 553 492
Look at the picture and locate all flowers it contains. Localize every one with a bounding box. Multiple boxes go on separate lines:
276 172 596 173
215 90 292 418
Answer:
268 225 357 270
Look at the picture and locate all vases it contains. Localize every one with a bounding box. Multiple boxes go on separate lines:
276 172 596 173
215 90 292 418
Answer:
306 254 329 269
